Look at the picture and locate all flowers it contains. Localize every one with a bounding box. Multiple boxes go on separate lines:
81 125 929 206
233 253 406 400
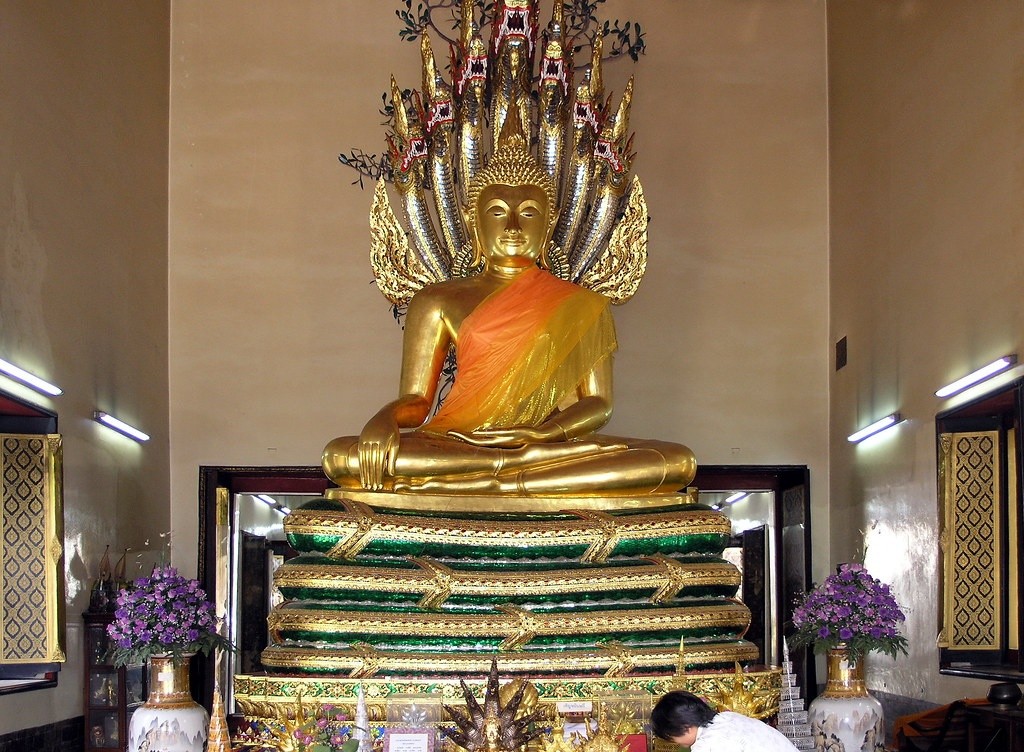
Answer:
786 563 910 668
100 560 241 667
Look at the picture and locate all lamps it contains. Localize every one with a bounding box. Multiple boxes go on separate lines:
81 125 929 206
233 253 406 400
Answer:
933 354 1017 398
0 358 64 397
94 410 151 442
847 414 900 443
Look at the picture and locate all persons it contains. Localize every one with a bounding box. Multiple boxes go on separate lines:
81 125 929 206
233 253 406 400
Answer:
317 134 701 502
472 718 514 752
646 689 806 752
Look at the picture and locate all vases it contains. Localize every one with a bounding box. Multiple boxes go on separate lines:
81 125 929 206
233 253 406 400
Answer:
129 651 210 752
809 643 886 752
